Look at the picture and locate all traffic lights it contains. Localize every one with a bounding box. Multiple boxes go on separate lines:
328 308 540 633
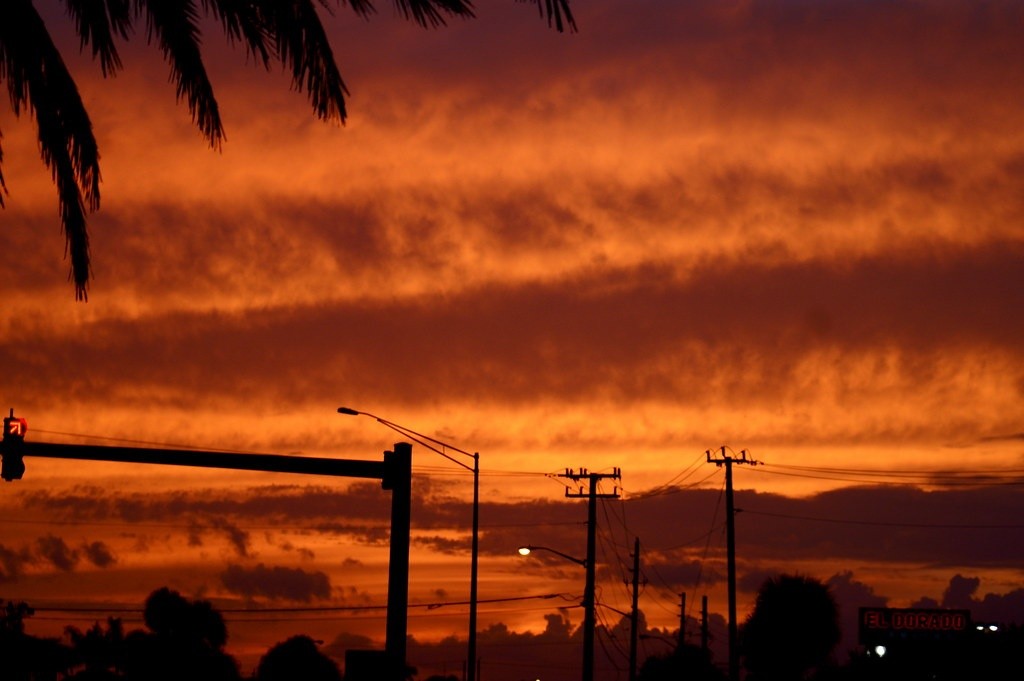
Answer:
3 417 26 480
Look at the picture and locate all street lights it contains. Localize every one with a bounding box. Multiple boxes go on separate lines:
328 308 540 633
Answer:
337 407 479 681
579 602 638 681
518 544 595 681
